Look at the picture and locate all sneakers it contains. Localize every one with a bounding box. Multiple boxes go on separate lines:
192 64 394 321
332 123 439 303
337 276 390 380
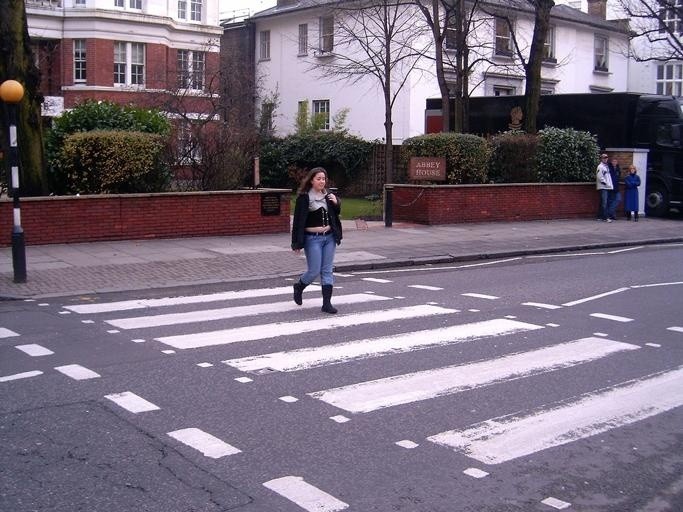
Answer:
598 215 638 223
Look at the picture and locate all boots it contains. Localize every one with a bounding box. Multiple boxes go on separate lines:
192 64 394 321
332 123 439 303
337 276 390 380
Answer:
293 277 308 305
321 285 337 313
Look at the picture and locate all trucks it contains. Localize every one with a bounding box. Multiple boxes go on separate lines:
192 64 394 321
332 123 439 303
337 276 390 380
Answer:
441 90 683 218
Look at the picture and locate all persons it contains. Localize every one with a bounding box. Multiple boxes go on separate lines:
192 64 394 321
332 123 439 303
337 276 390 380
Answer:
622 164 641 222
288 164 343 316
594 152 613 223
605 157 622 220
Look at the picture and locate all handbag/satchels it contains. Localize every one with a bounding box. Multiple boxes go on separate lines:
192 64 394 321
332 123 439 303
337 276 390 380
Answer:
332 216 342 240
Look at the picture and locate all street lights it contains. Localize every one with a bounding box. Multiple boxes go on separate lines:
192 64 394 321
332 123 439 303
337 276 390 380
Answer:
0 79 31 285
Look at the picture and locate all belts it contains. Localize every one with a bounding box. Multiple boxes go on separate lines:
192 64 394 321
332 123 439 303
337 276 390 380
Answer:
304 228 333 235
